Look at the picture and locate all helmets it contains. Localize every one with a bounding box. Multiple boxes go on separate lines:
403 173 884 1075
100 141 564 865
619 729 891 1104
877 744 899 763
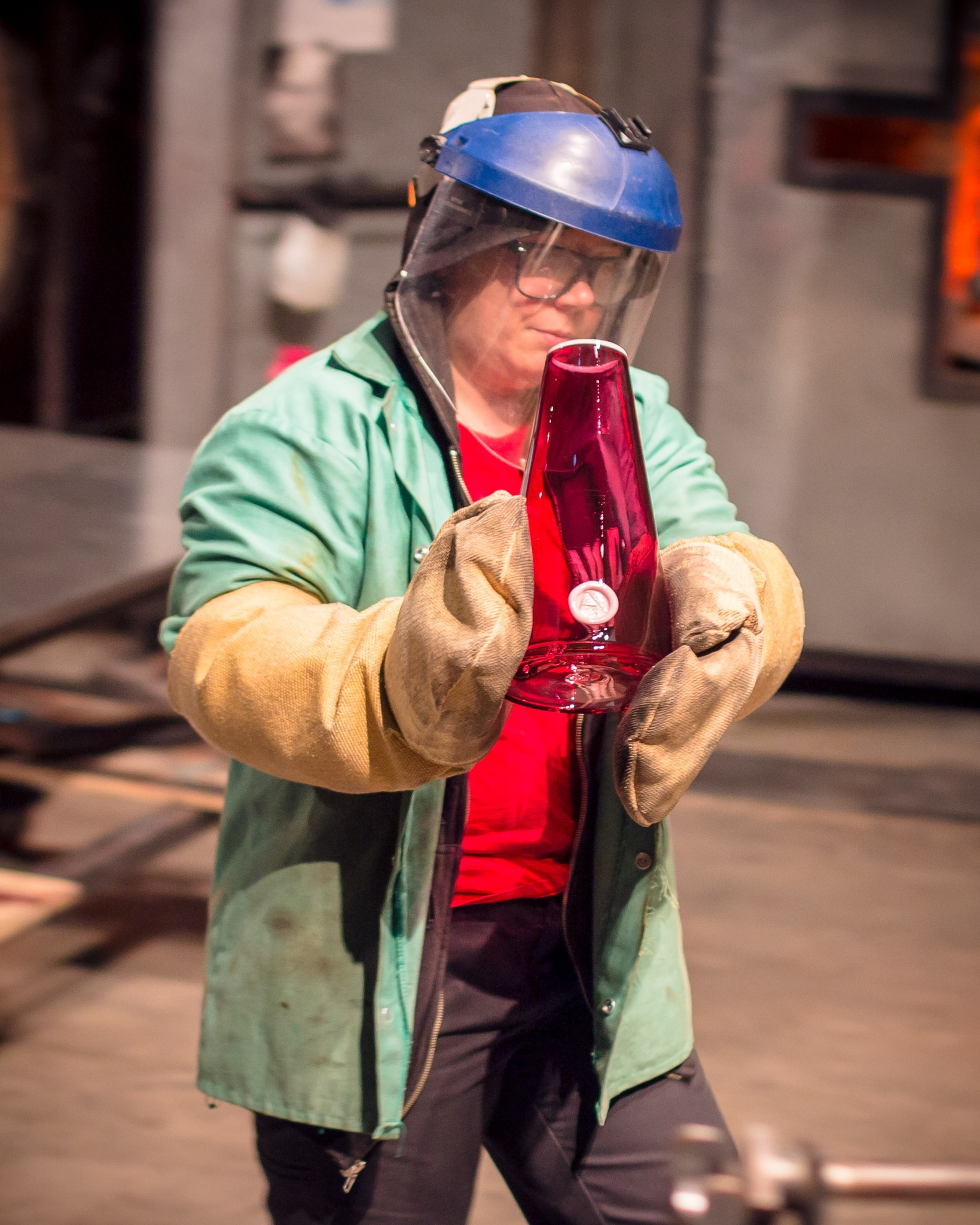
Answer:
419 75 682 252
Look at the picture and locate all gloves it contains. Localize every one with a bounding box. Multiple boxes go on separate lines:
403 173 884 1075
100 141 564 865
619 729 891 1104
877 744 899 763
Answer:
167 489 533 794
607 532 804 828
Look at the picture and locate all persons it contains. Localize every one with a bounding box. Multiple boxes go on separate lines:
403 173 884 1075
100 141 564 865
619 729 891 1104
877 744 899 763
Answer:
160 74 809 1225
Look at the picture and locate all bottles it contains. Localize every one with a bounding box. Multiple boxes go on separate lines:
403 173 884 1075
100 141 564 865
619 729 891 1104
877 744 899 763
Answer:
503 335 673 717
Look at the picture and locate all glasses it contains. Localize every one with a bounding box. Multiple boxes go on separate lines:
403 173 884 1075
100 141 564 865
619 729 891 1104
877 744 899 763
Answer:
502 240 652 306
